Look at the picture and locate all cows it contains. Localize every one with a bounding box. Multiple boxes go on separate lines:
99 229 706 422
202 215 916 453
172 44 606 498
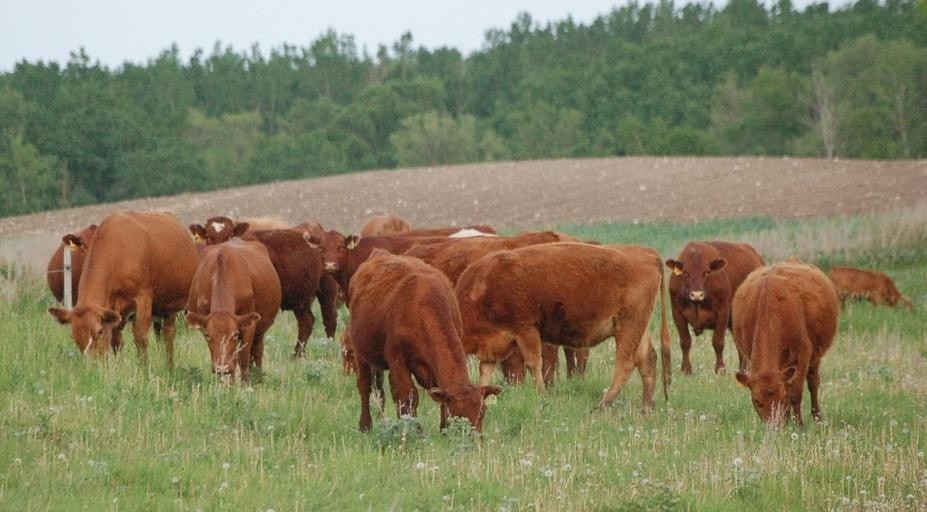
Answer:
665 239 767 375
831 266 915 313
45 210 673 449
730 255 841 428
